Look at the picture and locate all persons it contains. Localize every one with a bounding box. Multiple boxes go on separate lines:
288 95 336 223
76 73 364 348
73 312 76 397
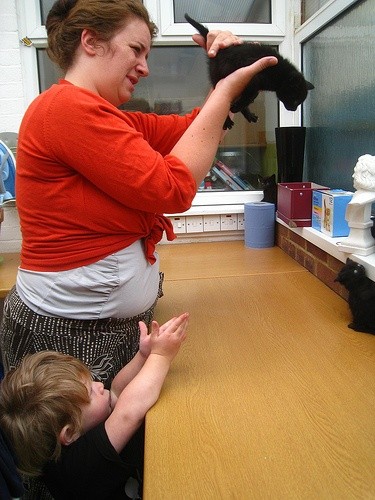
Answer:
0 0 280 500
0 312 192 500
344 154 375 224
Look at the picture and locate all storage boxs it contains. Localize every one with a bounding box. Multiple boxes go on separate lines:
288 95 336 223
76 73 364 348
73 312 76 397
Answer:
311 188 355 238
277 181 330 228
243 201 277 249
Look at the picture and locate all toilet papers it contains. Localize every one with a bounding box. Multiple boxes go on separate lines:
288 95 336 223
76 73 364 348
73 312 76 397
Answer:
243 201 277 249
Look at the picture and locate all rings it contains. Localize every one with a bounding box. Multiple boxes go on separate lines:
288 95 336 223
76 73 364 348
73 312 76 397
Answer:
233 36 239 40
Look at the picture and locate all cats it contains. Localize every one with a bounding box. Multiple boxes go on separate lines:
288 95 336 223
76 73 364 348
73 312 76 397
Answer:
184 12 315 130
258 174 278 211
333 257 375 336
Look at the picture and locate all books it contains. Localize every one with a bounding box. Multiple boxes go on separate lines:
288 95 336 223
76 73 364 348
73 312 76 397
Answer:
212 166 239 191
219 170 243 191
217 161 250 191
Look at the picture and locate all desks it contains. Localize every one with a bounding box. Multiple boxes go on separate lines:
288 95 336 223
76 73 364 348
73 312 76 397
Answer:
0 239 310 302
141 273 375 500
